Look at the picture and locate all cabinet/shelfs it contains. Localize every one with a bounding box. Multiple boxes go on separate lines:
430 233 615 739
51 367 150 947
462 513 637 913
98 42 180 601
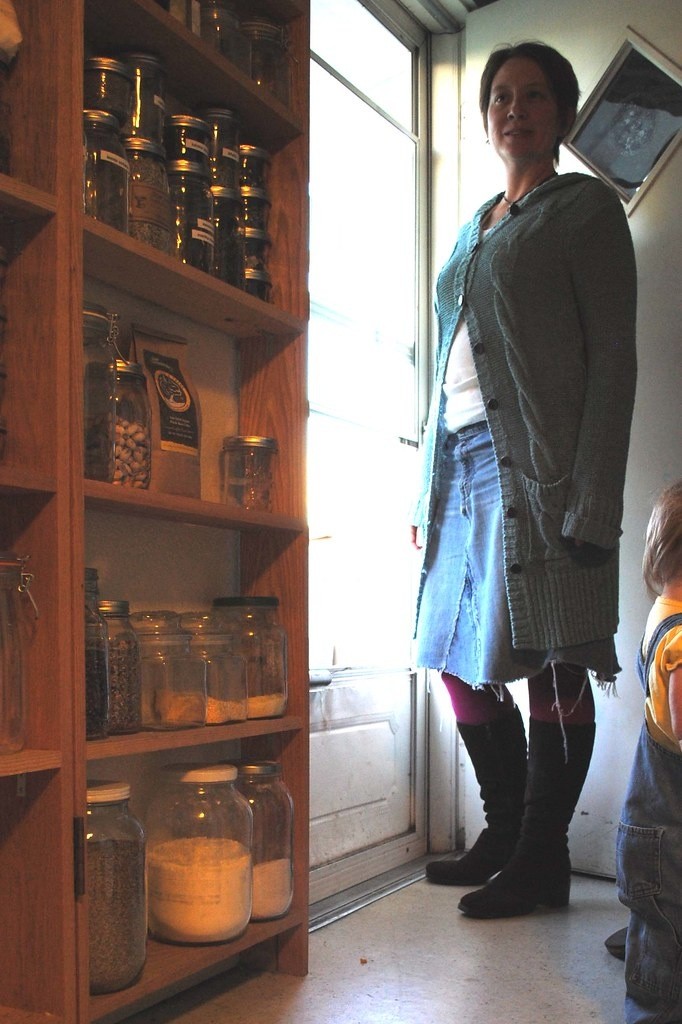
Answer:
71 0 308 1023
0 0 79 1024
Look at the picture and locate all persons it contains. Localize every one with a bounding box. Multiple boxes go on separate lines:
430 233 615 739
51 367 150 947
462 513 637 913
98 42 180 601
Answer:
616 484 682 1024
414 42 638 917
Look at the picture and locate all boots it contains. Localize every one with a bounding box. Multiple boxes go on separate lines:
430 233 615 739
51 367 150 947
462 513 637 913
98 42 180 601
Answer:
426 703 527 886
458 716 596 917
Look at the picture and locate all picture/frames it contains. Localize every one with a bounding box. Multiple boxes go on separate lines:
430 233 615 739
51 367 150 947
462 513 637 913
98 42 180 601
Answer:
561 25 682 217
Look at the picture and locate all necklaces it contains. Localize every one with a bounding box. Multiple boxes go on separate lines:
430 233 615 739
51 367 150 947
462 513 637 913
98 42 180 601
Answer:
502 171 558 203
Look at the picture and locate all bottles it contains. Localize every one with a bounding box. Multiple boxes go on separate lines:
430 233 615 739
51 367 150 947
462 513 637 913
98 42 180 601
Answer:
2 306 293 991
